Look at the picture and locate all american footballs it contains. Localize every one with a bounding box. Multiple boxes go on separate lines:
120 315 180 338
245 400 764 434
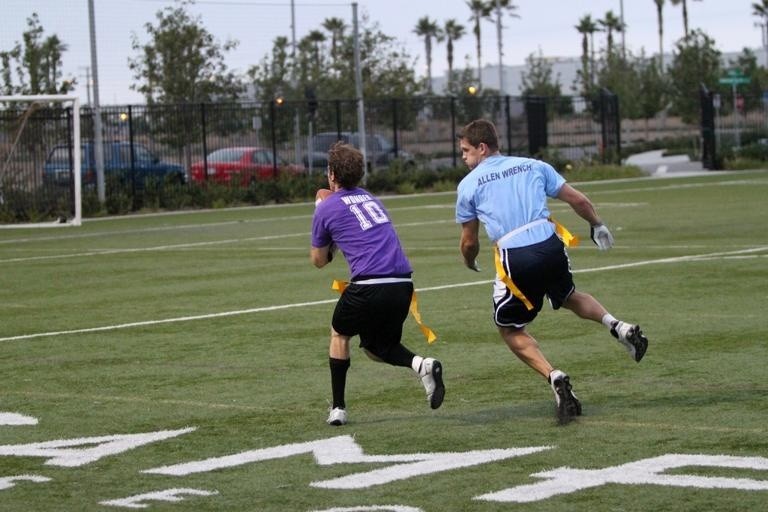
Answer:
315 189 332 207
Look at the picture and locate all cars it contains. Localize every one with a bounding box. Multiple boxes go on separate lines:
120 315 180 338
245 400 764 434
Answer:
190 146 307 188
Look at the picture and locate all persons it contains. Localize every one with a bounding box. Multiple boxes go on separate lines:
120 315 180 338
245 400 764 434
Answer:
310 140 445 427
454 120 647 418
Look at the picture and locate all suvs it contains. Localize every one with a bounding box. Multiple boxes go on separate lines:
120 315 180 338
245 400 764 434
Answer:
302 132 415 180
42 139 188 197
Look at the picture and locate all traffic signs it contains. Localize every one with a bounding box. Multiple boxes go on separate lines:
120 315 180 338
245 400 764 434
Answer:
724 67 744 76
717 76 751 85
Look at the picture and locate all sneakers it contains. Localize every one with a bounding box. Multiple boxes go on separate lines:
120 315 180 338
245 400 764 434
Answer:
324 396 347 426
613 320 649 363
548 368 583 416
417 358 446 410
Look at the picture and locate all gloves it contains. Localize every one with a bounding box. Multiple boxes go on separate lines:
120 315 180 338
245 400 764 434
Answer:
589 221 615 252
